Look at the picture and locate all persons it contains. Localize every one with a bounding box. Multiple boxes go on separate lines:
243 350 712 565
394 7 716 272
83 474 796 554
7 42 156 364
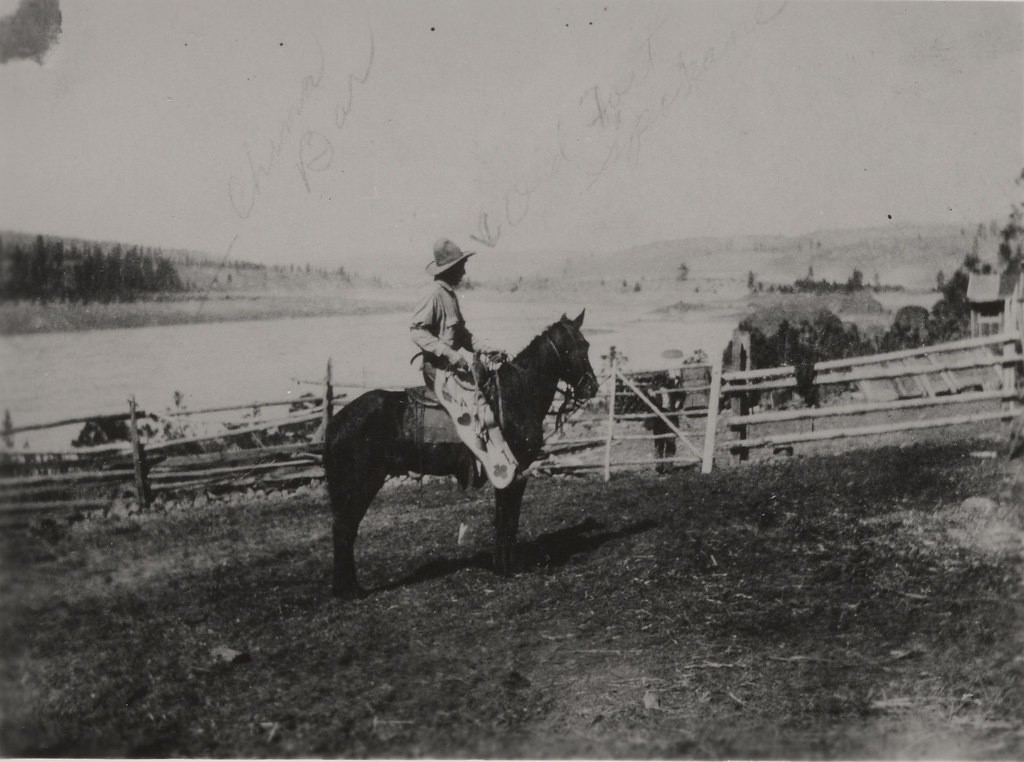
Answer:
409 238 519 490
643 369 687 473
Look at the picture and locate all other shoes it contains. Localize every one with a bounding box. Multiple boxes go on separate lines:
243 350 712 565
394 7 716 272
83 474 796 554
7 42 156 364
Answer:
665 469 672 474
657 466 663 473
457 467 487 490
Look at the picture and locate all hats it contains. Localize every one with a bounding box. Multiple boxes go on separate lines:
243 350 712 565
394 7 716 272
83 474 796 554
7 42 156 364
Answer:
426 237 476 275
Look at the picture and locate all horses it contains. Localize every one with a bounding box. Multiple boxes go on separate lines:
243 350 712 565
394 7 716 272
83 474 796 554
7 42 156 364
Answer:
329 306 601 591
